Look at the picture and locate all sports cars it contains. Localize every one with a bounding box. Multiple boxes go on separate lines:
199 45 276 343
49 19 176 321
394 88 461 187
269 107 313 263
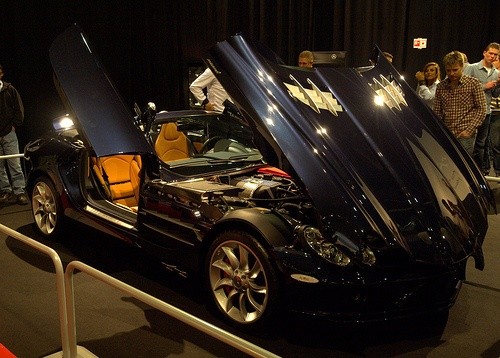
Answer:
22 21 498 332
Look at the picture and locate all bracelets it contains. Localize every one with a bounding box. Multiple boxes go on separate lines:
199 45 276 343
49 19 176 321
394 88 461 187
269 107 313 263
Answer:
201 104 206 110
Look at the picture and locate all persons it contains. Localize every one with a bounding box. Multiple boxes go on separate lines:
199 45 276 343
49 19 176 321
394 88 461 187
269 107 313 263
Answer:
444 42 500 177
185 68 235 114
415 62 441 112
382 52 393 63
434 51 487 157
0 64 29 205
298 50 313 68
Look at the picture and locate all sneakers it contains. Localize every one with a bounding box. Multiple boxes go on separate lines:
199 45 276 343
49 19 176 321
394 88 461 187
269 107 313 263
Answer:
17 194 29 204
0 192 14 202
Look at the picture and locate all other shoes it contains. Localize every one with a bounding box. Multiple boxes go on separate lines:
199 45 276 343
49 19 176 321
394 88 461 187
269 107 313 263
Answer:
484 173 489 176
496 173 500 183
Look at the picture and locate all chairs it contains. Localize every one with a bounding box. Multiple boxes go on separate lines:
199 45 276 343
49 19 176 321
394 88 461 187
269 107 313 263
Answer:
155 121 198 162
90 154 143 212
